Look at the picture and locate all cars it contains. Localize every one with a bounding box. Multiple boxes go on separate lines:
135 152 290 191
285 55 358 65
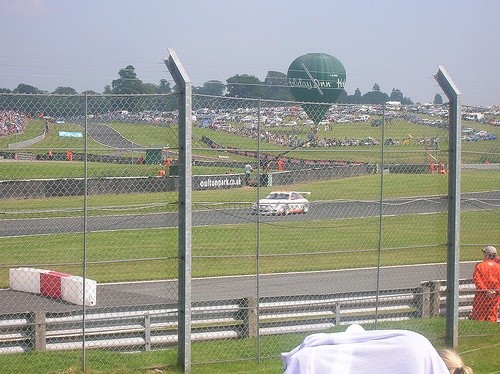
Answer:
252 191 311 215
40 101 500 172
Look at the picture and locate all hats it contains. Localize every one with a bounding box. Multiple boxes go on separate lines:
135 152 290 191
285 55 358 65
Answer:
482 246 497 256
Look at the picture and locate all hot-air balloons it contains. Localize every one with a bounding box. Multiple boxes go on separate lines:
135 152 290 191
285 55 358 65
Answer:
286 54 346 133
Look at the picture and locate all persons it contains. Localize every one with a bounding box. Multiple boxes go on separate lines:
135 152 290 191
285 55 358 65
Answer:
244 162 253 184
430 162 447 174
277 158 283 171
468 246 500 322
48 150 54 160
163 156 171 166
45 123 50 134
66 150 73 161
157 169 167 177
66 96 499 148
436 348 474 374
259 159 270 173
0 110 25 136
